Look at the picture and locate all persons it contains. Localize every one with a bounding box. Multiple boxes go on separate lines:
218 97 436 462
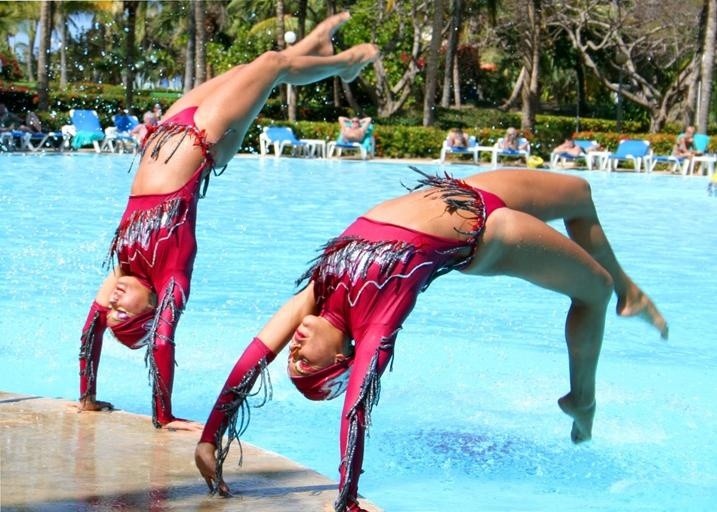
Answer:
0 102 163 153
586 142 606 154
497 128 532 150
444 125 470 150
552 138 581 159
62 9 382 435
336 115 372 144
192 166 669 511
671 126 701 175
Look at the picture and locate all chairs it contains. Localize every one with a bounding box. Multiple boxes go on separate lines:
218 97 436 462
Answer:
257 117 375 160
438 133 717 176
0 99 138 159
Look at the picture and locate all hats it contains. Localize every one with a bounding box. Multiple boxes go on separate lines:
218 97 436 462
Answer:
286 352 353 401
109 308 157 349
